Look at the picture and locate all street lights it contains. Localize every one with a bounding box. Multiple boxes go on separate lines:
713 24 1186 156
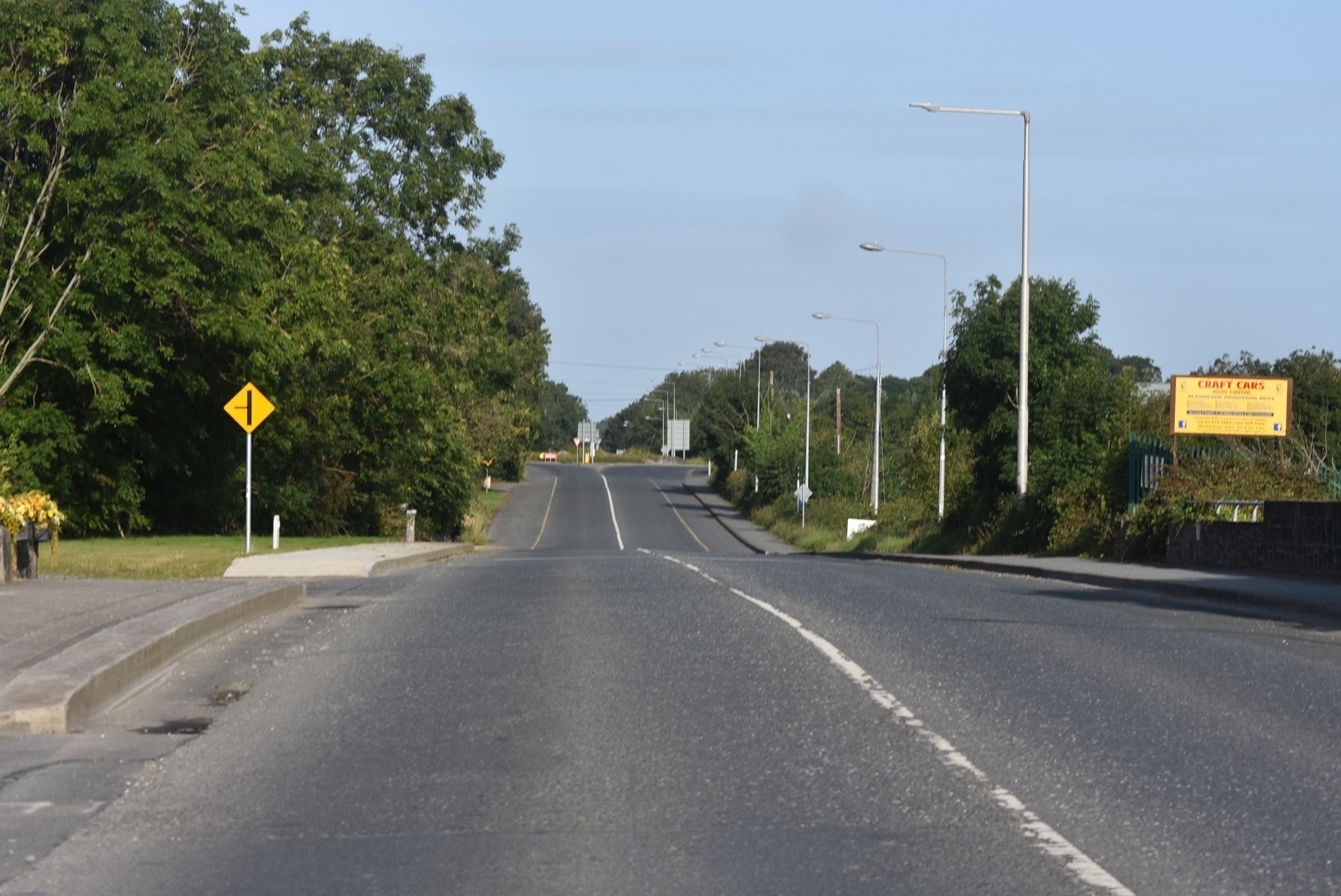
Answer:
644 380 676 458
910 101 1032 496
858 243 948 519
678 348 743 474
813 313 881 518
715 340 761 430
755 336 810 503
